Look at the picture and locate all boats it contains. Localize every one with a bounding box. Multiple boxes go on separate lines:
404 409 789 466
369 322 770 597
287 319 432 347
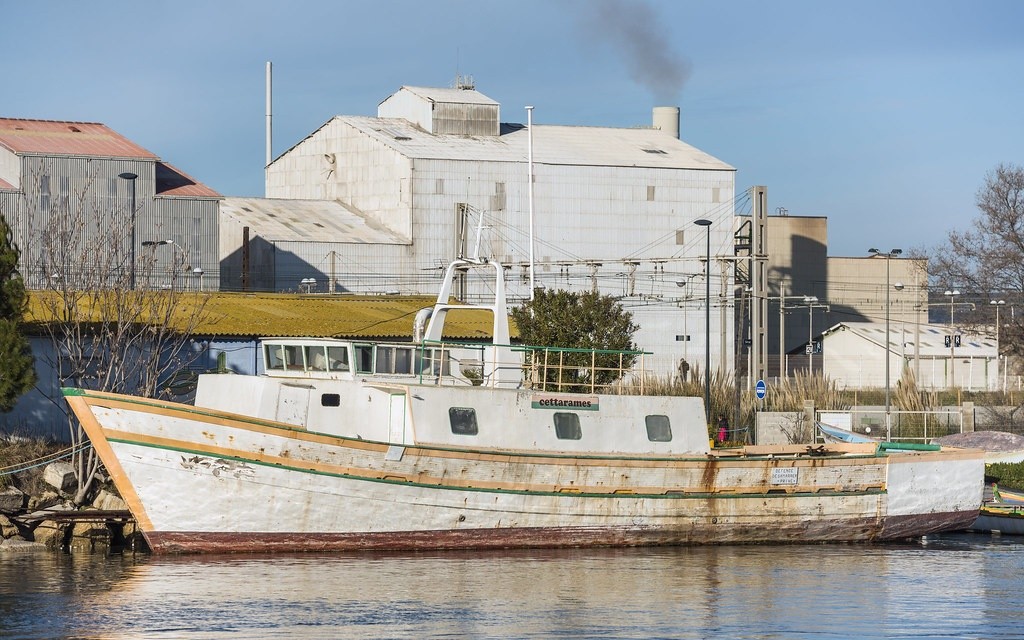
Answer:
58 209 989 556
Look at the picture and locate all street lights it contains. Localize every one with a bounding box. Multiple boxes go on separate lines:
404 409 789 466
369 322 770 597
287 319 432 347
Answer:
141 240 167 288
692 219 712 421
943 290 961 389
868 248 903 439
990 300 1005 388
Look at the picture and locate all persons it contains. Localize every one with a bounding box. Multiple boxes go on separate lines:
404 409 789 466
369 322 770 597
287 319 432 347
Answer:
678 358 689 381
992 483 1001 503
717 416 729 443
